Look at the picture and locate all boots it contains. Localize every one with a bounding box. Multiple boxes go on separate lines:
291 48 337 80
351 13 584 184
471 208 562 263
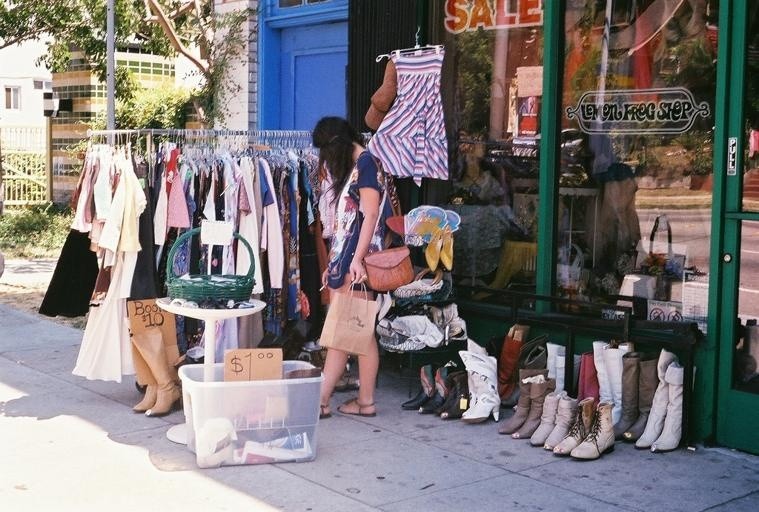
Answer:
127 328 184 420
497 325 684 460
402 337 501 423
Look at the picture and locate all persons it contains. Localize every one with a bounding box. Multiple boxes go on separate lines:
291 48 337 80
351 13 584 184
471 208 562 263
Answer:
445 131 506 278
575 135 642 305
307 116 403 420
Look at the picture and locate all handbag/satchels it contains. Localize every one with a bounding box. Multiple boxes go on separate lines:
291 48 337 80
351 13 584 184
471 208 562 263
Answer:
554 241 593 307
362 243 414 292
316 276 382 358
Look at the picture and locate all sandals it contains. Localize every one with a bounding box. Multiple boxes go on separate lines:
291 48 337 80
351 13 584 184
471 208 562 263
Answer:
337 398 377 418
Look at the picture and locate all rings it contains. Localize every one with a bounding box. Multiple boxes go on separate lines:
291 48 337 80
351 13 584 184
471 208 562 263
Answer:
360 274 368 281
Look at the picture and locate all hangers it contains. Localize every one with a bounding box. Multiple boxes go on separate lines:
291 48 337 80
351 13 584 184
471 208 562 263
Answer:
485 139 579 160
376 22 450 59
83 126 325 181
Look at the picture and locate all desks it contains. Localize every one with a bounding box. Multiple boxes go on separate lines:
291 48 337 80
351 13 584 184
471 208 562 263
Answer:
153 295 268 447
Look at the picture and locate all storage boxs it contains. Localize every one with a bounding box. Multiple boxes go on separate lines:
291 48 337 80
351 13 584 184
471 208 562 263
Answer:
178 355 324 468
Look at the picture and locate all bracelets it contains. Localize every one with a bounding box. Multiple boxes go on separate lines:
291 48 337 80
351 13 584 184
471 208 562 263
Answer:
353 253 365 260
